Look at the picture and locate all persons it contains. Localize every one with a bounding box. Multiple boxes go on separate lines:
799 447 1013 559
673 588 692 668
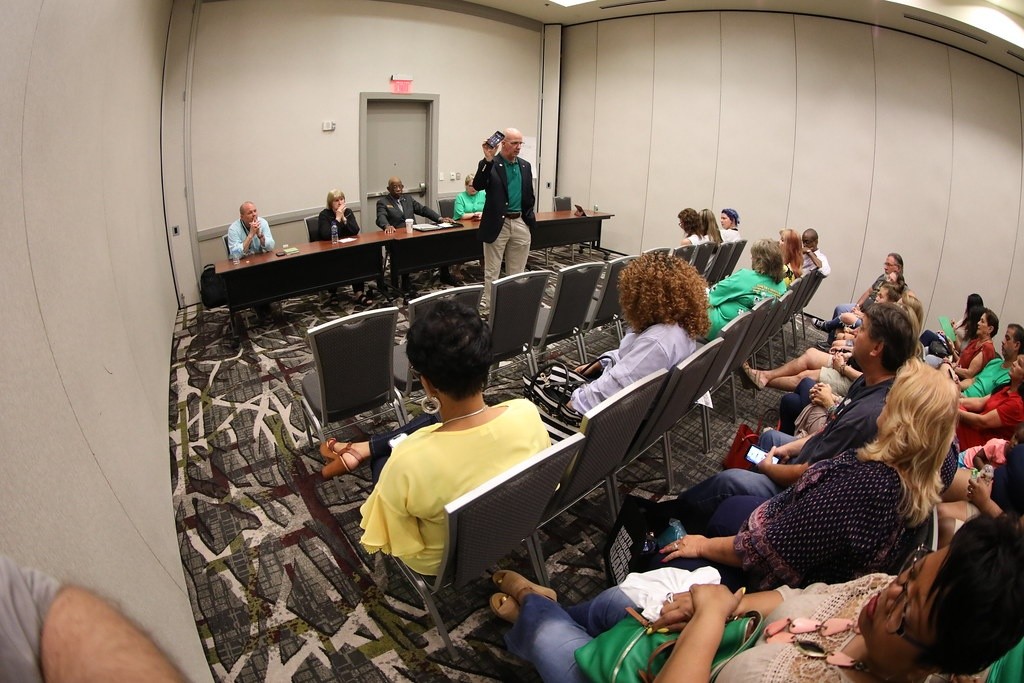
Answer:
622 252 961 594
453 174 487 281
566 256 712 416
675 208 741 244
318 189 374 307
488 516 1024 683
472 127 537 326
698 238 788 341
226 200 279 322
923 295 1024 682
1 548 196 683
777 228 831 288
376 176 462 294
319 298 554 577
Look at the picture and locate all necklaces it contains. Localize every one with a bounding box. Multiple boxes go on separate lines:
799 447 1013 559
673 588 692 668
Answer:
442 405 490 423
762 615 896 683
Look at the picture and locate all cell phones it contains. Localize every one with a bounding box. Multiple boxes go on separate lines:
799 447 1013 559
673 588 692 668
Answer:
948 369 954 380
276 252 285 256
744 444 781 466
388 433 408 448
485 131 505 149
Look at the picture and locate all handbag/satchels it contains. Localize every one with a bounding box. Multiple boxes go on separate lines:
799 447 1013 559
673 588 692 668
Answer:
603 494 670 588
522 355 616 446
722 409 781 470
574 607 766 682
928 340 950 359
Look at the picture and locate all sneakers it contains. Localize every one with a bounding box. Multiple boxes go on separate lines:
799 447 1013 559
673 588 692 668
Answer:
817 340 832 352
811 318 831 334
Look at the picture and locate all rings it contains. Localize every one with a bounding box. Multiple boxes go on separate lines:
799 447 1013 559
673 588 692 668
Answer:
672 541 679 550
666 592 674 603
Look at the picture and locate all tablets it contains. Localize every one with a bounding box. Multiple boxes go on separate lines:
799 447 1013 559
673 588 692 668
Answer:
574 205 587 216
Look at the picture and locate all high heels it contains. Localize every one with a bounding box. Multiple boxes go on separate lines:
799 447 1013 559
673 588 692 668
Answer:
320 438 365 480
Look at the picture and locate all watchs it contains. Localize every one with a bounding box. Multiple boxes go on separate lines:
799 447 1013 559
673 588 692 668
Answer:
258 231 265 239
839 362 848 375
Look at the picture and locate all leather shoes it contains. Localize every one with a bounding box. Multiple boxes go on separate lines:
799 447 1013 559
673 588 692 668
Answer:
440 274 460 286
402 283 416 293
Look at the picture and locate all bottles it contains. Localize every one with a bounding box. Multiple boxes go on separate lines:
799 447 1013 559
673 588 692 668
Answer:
232 244 240 265
331 222 339 244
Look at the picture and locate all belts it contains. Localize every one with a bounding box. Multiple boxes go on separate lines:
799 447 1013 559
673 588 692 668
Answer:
506 212 521 219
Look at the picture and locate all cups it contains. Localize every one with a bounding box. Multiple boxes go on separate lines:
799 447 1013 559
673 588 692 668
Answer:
405 219 414 234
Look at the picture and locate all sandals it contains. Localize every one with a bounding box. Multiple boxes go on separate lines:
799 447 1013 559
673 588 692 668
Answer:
490 570 557 625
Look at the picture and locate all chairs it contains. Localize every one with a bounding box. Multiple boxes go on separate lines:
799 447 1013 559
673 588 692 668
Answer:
298 238 826 662
304 215 320 242
436 196 456 220
553 197 592 263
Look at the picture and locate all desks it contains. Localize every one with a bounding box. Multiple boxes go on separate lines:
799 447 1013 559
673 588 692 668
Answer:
214 208 616 348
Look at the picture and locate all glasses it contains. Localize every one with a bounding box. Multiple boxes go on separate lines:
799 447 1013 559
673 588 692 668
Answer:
803 237 818 243
885 544 935 649
469 185 474 189
678 222 684 227
410 365 423 382
511 142 526 147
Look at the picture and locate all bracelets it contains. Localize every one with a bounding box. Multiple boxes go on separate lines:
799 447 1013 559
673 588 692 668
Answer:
824 402 841 421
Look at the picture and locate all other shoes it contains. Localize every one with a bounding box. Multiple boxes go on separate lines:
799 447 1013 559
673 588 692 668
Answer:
739 361 769 391
449 270 465 280
258 313 275 324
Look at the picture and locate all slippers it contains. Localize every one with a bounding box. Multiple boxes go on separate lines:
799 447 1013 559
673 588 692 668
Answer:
354 294 373 306
329 295 339 305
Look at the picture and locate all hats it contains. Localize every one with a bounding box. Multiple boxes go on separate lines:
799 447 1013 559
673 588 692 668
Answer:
722 208 740 224
